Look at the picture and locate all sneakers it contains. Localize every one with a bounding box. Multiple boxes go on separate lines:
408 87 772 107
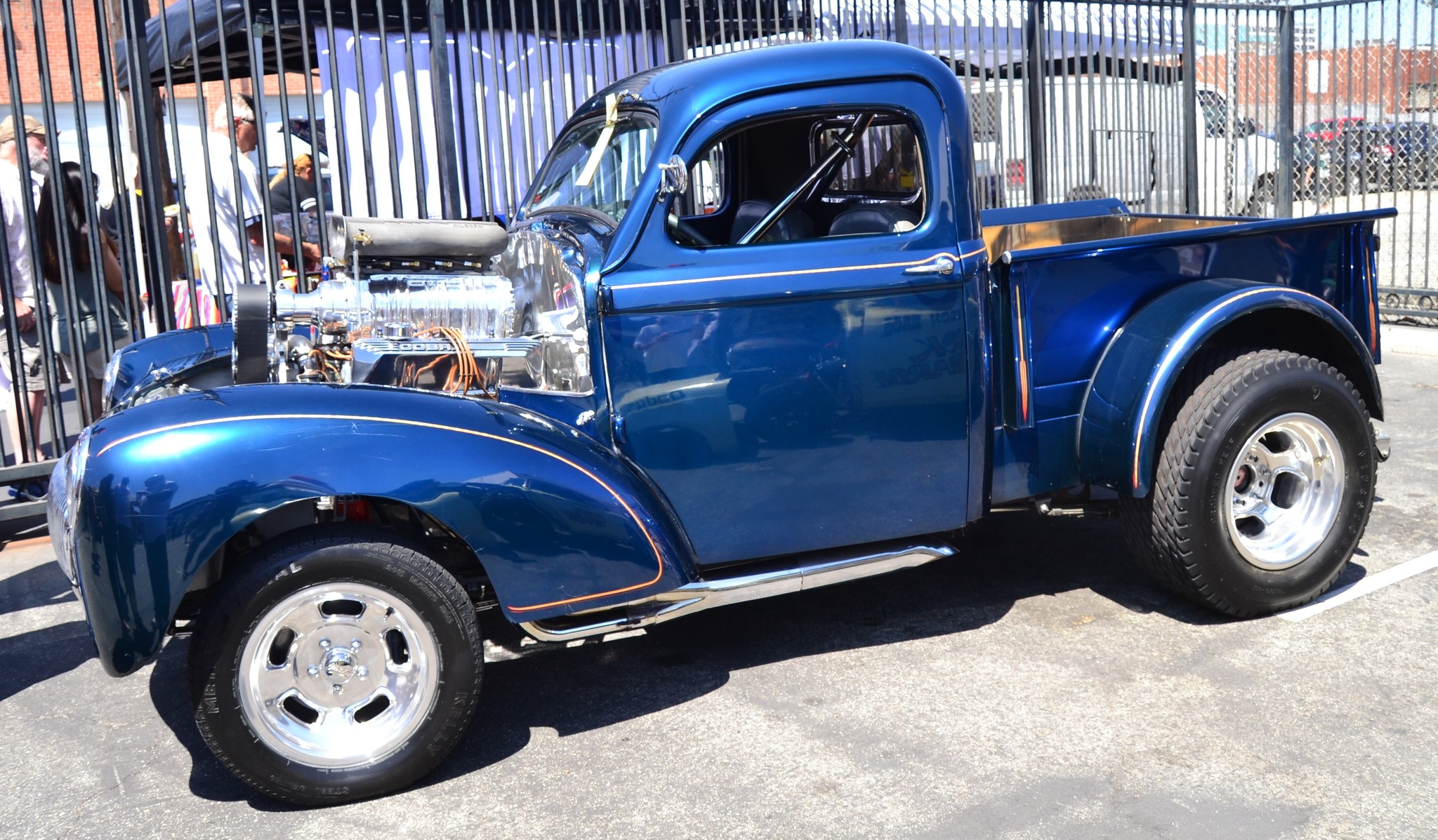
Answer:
8 455 54 502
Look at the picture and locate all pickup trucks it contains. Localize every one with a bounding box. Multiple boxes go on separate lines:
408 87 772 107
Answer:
48 40 1398 809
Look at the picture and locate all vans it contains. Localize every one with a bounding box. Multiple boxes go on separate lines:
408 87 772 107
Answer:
947 74 1280 220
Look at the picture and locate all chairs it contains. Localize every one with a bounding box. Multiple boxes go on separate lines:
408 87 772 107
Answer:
829 206 918 235
730 199 811 243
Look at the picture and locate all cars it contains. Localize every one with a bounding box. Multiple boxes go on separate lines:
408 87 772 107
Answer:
1211 117 1437 197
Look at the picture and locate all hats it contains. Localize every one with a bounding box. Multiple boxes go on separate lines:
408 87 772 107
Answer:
0 114 61 143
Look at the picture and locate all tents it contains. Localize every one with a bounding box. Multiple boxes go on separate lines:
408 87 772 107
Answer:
787 0 1204 209
112 0 817 333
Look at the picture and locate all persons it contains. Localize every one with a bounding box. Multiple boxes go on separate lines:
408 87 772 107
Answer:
0 93 320 503
70 474 181 663
